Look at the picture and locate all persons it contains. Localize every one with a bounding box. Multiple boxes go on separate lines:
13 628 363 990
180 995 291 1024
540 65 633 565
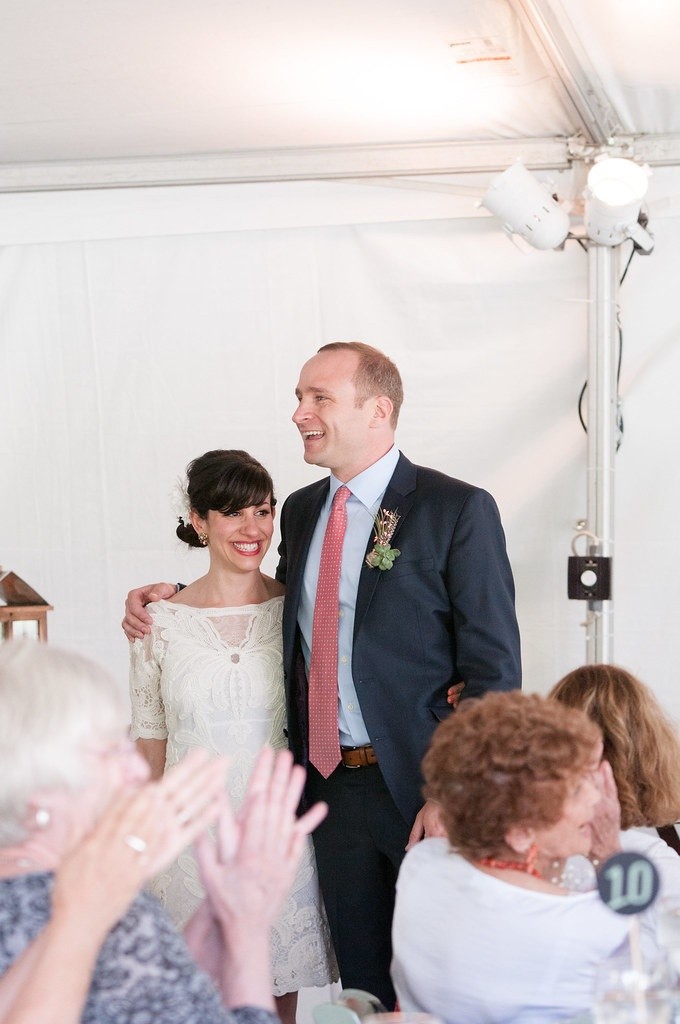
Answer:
393 666 679 1024
121 339 522 1011
1 639 329 1024
129 447 464 1024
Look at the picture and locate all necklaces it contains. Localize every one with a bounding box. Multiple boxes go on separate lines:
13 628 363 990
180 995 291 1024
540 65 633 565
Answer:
482 858 544 879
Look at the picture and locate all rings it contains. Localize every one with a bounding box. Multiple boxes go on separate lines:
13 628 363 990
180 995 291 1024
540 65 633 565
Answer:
125 833 146 852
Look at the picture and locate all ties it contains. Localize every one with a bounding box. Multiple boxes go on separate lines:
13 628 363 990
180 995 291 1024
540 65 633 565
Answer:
307 485 355 778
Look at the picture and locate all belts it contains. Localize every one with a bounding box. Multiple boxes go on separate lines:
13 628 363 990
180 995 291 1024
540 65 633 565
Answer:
334 746 376 768
